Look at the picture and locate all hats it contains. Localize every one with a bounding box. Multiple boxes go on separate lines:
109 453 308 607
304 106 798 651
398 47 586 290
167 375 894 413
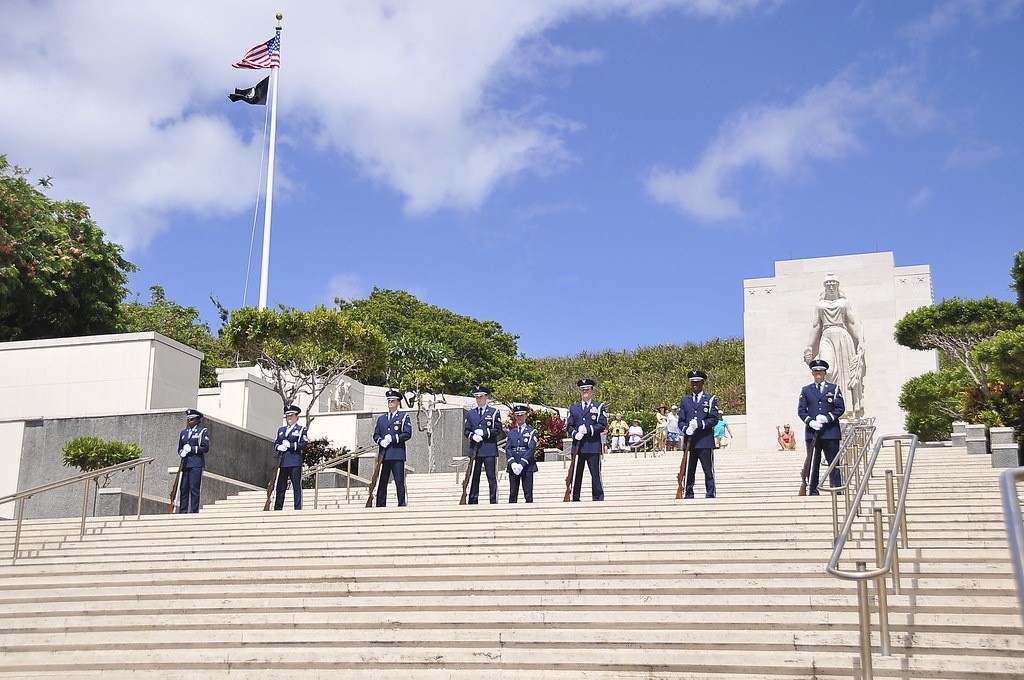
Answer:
632 419 640 423
386 391 403 401
784 424 790 427
809 359 829 371
472 386 491 398
512 406 529 416
284 406 301 415
186 409 203 419
671 405 677 410
718 410 724 414
577 379 596 389
687 370 707 382
658 404 667 411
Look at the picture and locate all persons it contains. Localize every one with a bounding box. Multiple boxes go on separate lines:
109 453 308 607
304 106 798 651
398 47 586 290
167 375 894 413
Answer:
463 386 502 504
567 379 608 502
776 423 796 451
628 419 643 442
373 390 411 507
797 360 845 496
274 405 307 511
655 404 668 451
678 370 719 499
803 272 867 419
667 405 680 451
609 413 629 449
178 408 209 514
713 410 733 449
505 405 538 503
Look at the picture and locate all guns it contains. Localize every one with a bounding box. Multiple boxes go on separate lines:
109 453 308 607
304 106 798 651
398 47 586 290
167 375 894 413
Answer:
675 435 692 499
263 452 283 511
798 431 819 496
365 447 386 507
563 440 580 502
459 443 479 505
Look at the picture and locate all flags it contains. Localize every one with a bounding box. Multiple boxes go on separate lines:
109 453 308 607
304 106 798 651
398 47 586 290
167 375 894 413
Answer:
231 30 281 70
228 75 270 106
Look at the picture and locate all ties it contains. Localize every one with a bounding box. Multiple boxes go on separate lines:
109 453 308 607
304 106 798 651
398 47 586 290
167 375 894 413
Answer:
695 393 698 402
818 383 821 393
518 426 521 433
389 413 393 420
479 407 483 417
584 402 588 410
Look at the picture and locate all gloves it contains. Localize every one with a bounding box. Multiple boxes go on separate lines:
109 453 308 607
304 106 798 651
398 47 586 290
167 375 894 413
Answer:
685 419 698 435
472 429 483 442
574 425 587 440
380 434 392 448
810 414 828 430
276 440 290 452
180 444 191 457
511 462 524 475
